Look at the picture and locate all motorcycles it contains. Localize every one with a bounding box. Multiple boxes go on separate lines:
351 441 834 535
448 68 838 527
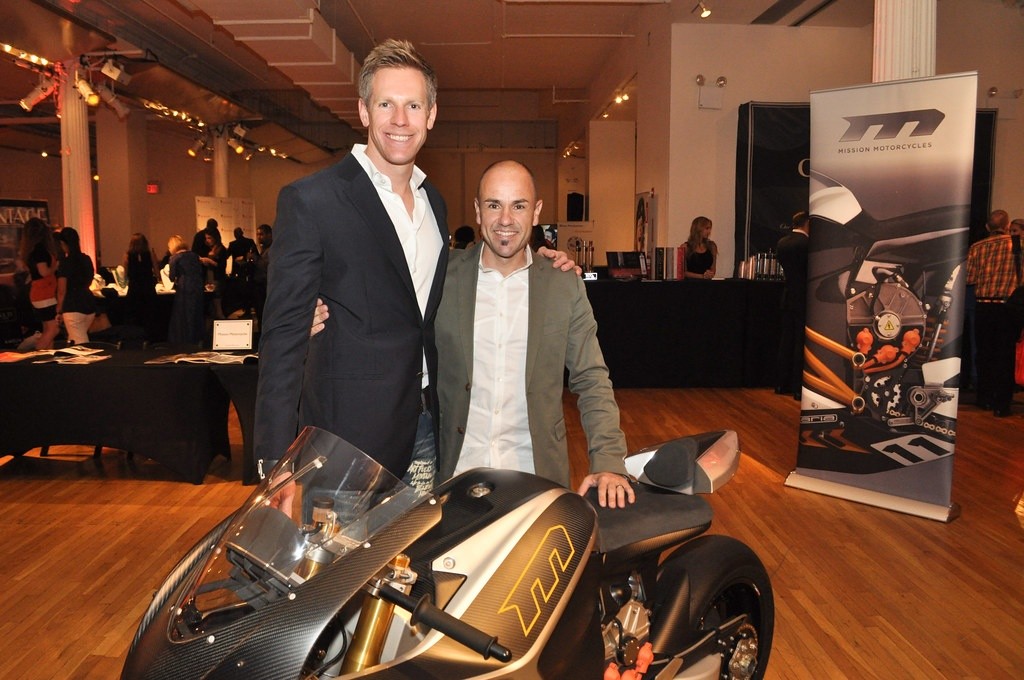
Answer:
124 427 778 680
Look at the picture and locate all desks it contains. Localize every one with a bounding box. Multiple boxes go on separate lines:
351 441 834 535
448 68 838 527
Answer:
575 277 803 389
0 345 259 484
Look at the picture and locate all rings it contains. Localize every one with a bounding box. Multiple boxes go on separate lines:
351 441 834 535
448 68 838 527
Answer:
616 485 624 489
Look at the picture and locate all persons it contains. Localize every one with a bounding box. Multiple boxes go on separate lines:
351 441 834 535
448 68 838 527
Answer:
0 217 273 354
680 217 718 280
310 159 636 509
966 210 1024 417
775 211 809 400
452 226 476 249
253 38 450 519
544 229 556 243
529 224 548 252
1010 219 1024 235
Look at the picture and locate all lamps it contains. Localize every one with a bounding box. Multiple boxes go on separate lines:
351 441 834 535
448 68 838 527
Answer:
699 1 711 19
696 74 726 110
189 138 214 161
227 139 243 154
562 91 631 158
99 83 130 117
20 79 54 111
76 78 98 105
